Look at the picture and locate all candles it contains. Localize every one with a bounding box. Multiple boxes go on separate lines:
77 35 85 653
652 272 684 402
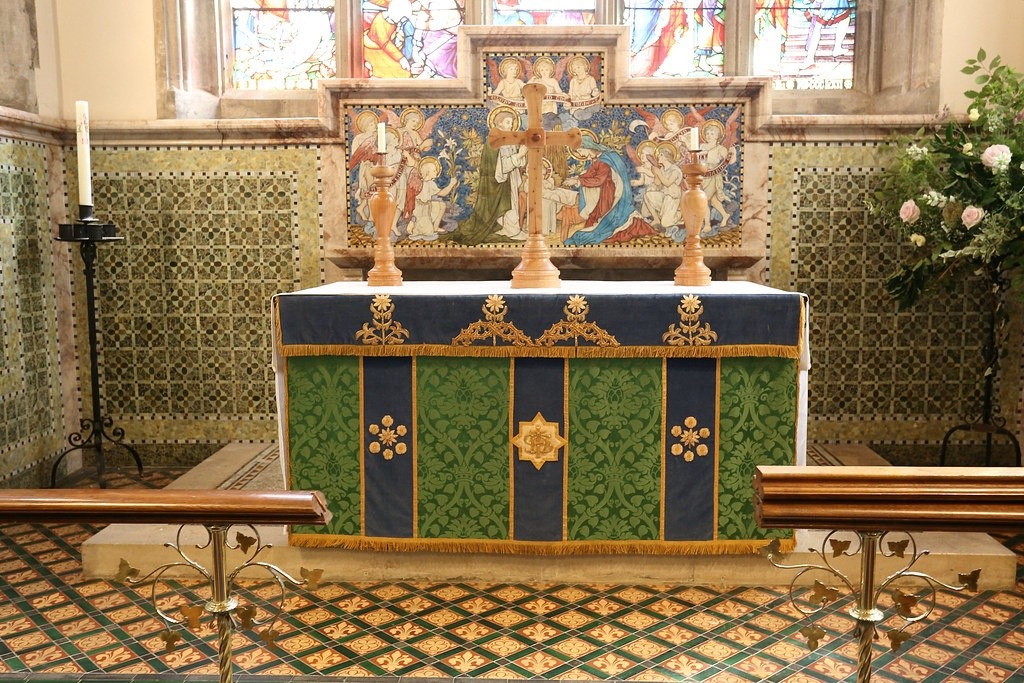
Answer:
76 101 91 206
378 122 386 152
691 126 699 151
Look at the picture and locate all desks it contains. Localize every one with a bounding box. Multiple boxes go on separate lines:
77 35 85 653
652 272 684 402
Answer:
269 279 810 556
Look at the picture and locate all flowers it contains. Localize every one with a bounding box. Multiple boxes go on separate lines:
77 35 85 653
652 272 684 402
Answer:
861 46 1024 316
